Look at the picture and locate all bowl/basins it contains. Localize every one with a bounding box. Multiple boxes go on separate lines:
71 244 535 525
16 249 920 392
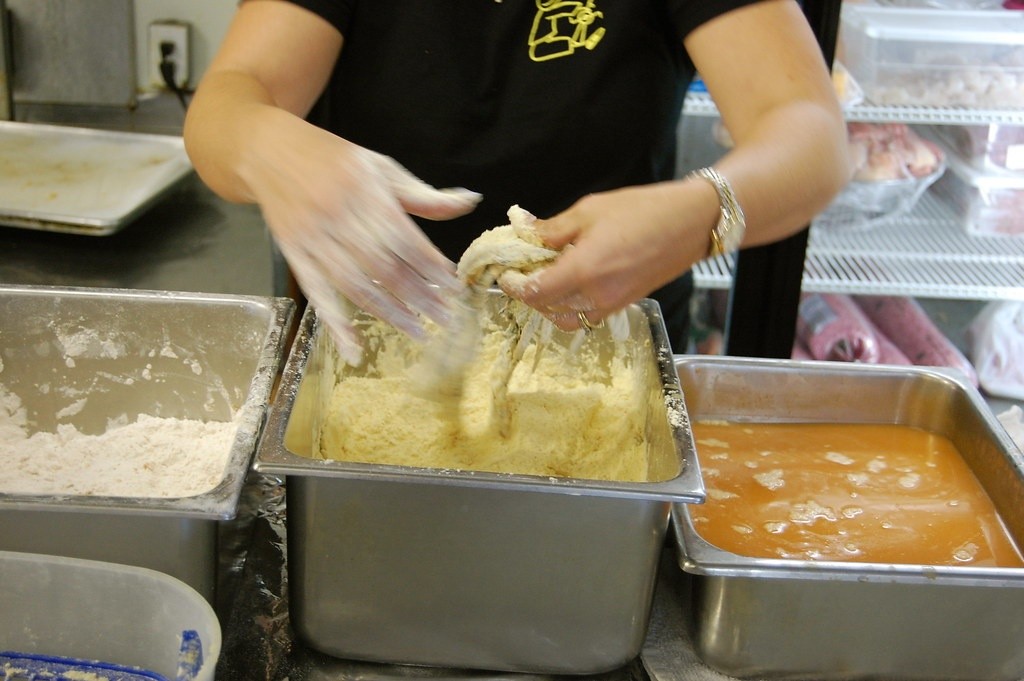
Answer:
710 117 947 230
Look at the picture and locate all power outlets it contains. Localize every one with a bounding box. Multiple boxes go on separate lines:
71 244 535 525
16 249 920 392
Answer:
149 19 189 87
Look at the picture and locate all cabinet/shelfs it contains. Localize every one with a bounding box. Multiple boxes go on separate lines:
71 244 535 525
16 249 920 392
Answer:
678 91 1024 301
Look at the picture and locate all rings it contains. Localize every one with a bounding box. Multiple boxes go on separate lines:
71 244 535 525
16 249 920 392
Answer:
577 311 605 335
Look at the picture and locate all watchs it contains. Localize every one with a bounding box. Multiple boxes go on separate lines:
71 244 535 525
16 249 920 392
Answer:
686 167 746 257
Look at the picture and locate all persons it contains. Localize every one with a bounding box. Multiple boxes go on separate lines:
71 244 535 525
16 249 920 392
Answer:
182 0 850 367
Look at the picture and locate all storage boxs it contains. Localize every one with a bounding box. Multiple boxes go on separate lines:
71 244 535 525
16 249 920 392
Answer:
0 549 221 681
836 0 1024 241
